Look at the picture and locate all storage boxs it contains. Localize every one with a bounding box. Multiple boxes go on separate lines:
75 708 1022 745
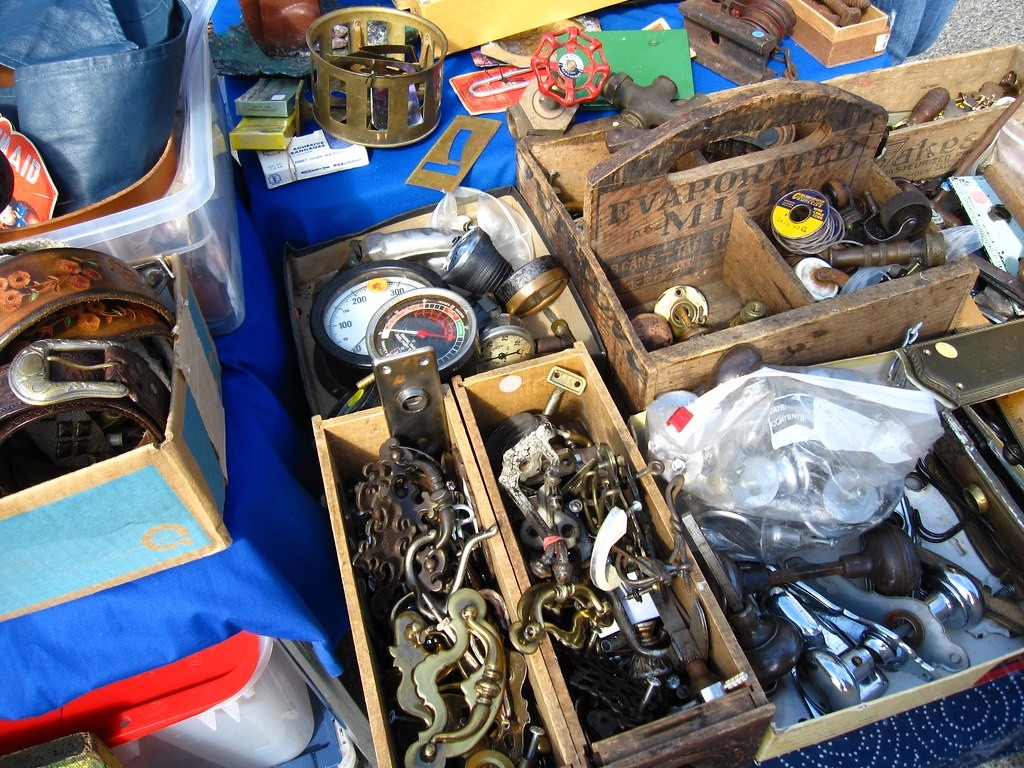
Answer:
787 16 891 68
626 351 1024 762
229 110 296 150
820 44 1024 181
235 78 297 118
0 27 247 339
786 0 888 43
408 0 626 58
258 129 370 189
516 76 978 413
0 630 315 768
0 252 235 621
449 340 775 768
310 382 581 768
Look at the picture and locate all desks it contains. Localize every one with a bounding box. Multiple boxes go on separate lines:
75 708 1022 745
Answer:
0 160 377 768
208 0 893 284
